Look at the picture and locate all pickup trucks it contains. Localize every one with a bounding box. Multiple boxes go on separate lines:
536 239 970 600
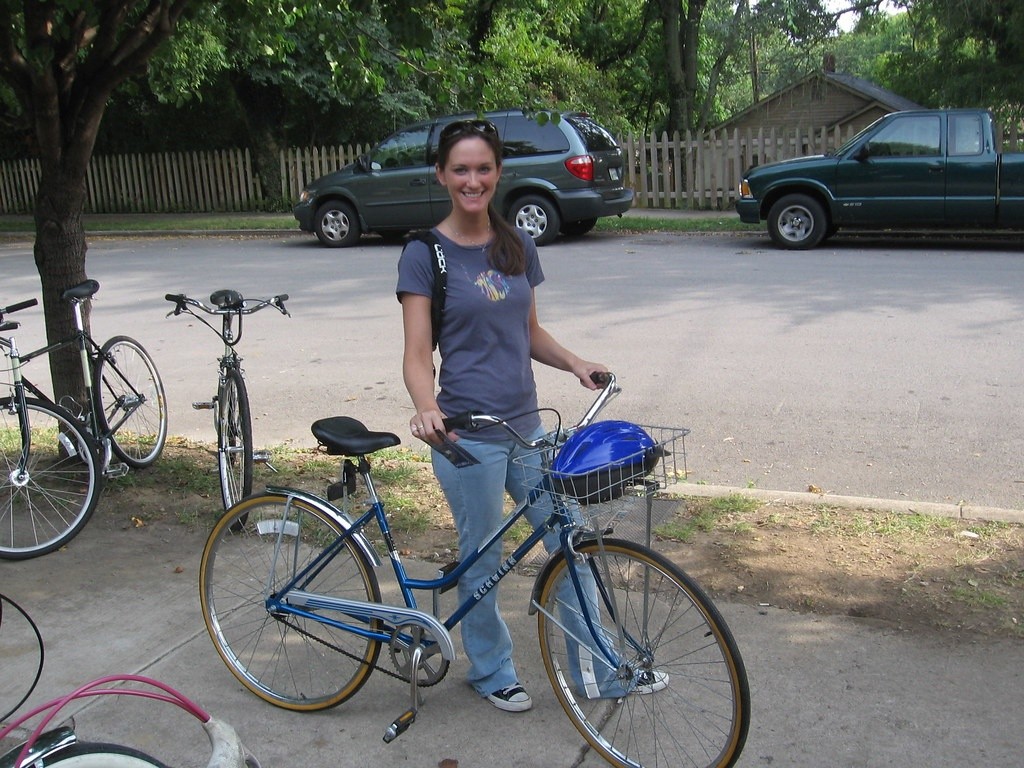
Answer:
734 108 1023 250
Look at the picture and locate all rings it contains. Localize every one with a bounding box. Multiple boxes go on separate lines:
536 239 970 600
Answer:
418 426 423 430
410 425 417 434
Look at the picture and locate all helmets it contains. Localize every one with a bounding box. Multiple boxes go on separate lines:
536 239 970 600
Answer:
551 421 663 502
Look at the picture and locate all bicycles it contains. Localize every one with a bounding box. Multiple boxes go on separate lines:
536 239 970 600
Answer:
0 280 168 563
197 370 751 768
162 287 292 531
0 592 264 768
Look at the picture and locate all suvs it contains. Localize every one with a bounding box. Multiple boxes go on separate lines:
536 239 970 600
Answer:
293 109 634 248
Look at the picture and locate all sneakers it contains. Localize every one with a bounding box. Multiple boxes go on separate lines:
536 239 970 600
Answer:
632 671 669 695
486 682 531 712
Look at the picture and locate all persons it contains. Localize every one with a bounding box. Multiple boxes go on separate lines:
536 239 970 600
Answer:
397 118 670 712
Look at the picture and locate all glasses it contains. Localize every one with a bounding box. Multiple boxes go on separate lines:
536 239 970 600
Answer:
438 120 499 150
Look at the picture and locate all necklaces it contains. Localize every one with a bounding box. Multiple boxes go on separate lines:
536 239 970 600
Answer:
448 215 490 253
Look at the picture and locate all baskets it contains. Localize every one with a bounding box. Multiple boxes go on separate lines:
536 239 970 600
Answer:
512 422 690 522
